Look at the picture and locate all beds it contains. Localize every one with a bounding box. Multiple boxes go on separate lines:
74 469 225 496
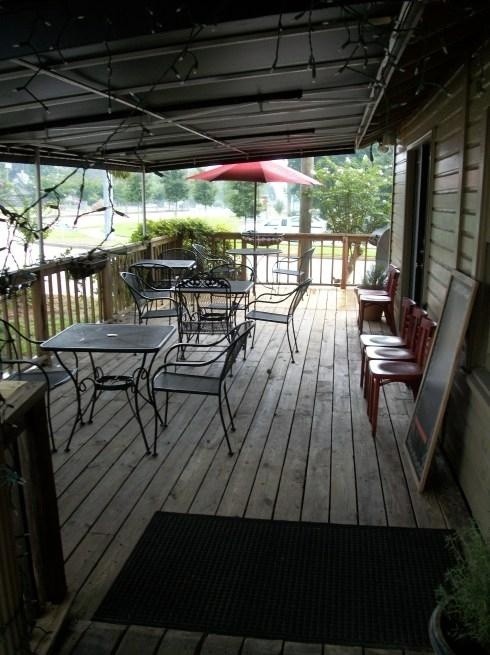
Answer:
184 159 324 252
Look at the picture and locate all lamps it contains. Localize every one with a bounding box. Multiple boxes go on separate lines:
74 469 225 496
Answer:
357 264 401 333
361 297 440 437
1 320 78 453
118 271 193 358
275 249 313 302
193 243 227 287
150 321 254 457
243 279 308 364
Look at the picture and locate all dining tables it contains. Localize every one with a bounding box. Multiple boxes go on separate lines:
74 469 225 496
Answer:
425 527 490 655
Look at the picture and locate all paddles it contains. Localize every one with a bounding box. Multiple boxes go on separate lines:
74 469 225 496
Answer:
95 511 469 650
